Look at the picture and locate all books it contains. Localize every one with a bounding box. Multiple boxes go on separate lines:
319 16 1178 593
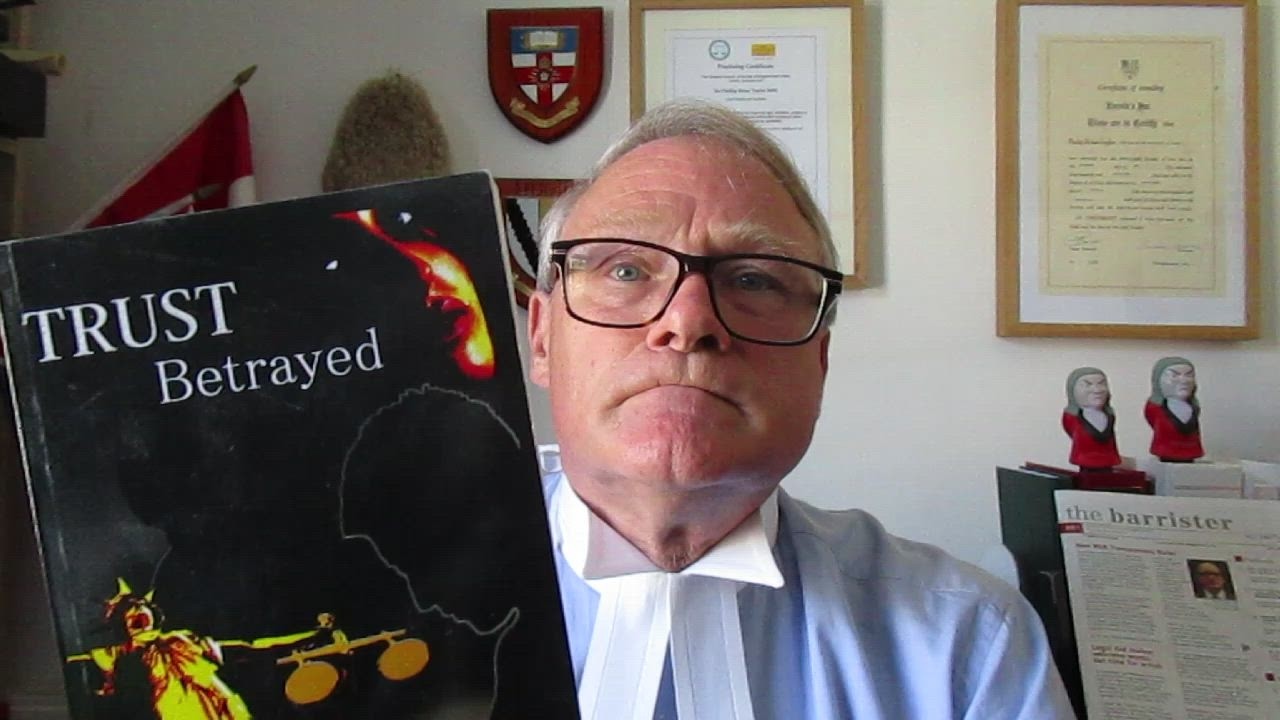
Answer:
0 170 585 720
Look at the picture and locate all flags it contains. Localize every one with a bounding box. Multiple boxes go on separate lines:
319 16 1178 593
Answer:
62 76 257 237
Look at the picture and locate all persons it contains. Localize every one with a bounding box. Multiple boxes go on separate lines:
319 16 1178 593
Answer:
1196 562 1235 599
1063 368 1123 473
526 100 1080 720
1144 358 1205 463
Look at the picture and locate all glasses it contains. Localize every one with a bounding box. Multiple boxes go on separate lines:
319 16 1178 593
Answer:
546 238 847 347
